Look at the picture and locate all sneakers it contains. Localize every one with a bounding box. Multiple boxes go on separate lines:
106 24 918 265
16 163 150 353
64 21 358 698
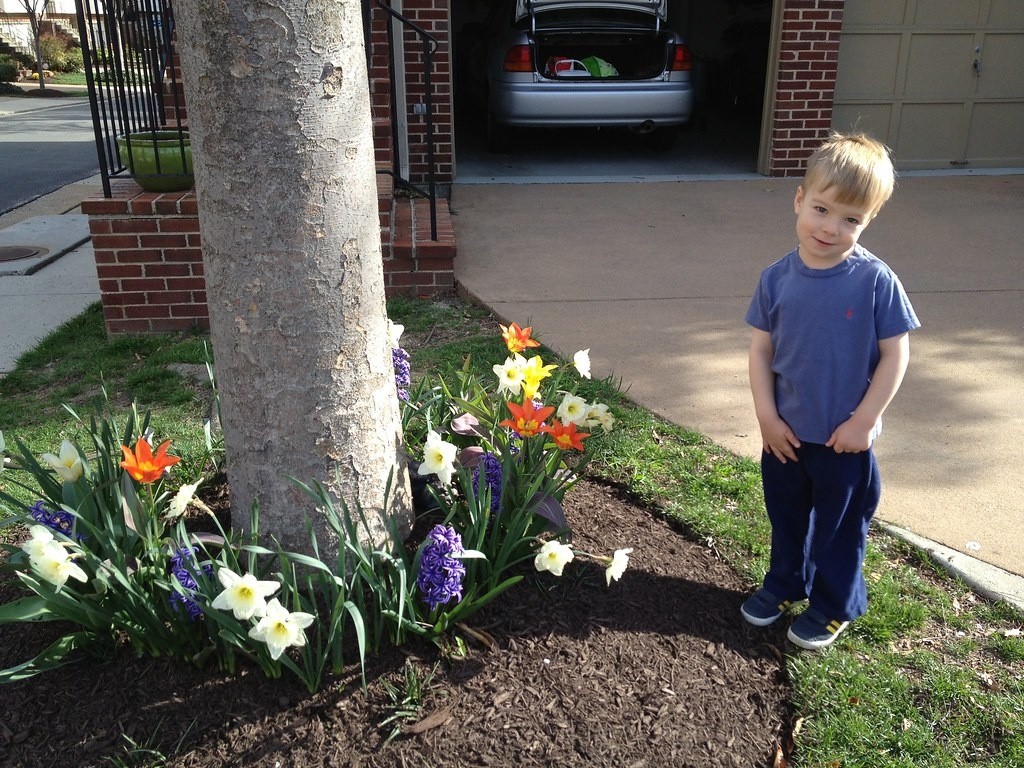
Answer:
740 579 810 626
788 604 851 650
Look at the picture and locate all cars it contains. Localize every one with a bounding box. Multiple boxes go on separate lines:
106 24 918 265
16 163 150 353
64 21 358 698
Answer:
485 0 704 136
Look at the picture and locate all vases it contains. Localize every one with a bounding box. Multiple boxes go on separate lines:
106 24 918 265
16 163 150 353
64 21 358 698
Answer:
117 130 195 191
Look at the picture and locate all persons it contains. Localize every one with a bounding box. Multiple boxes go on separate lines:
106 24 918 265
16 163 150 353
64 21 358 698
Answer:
740 134 922 649
